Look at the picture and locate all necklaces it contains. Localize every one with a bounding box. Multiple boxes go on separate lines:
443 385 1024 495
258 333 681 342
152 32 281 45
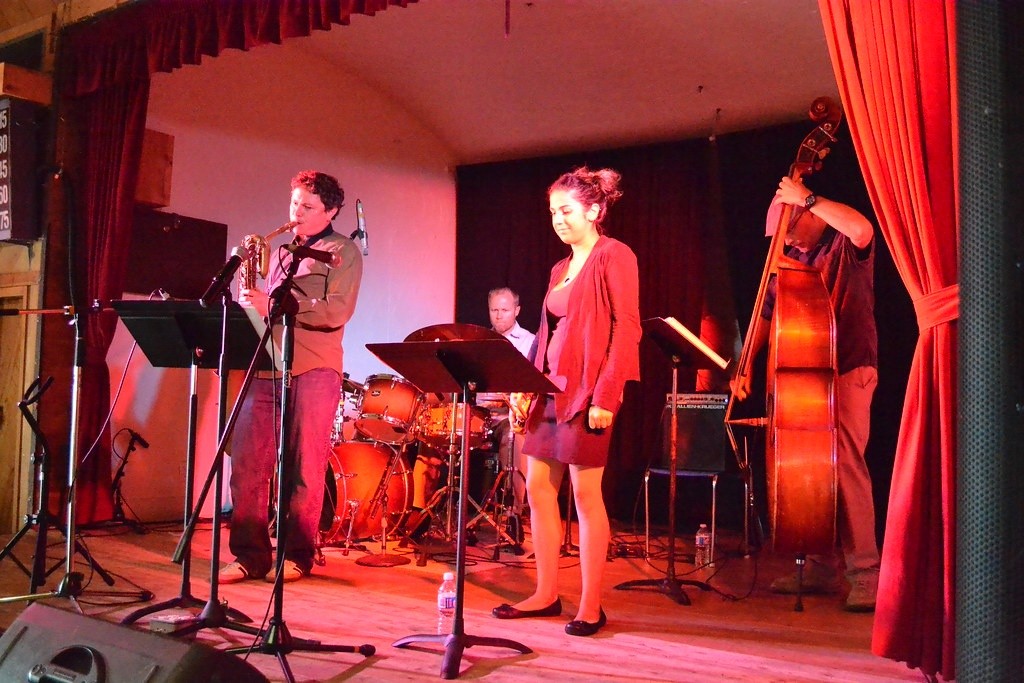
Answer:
564 277 571 283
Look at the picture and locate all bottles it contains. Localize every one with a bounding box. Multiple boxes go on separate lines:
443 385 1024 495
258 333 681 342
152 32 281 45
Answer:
437 573 456 635
695 524 710 568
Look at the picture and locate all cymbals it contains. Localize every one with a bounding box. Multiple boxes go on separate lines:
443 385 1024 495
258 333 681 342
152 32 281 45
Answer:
340 377 364 393
403 321 513 343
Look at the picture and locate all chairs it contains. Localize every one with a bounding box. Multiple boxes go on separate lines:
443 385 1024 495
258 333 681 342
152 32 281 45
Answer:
644 391 749 566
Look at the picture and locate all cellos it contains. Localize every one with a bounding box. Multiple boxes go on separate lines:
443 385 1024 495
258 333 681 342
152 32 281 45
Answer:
765 95 846 615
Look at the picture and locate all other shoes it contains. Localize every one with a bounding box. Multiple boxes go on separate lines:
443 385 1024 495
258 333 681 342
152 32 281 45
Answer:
492 596 565 619
565 604 607 636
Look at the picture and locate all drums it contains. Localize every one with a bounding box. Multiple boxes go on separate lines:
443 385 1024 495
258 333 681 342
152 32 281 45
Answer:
412 442 449 510
353 373 424 446
316 438 416 543
424 402 491 448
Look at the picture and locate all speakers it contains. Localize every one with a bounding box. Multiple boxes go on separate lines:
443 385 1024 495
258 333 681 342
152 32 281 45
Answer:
0 601 271 683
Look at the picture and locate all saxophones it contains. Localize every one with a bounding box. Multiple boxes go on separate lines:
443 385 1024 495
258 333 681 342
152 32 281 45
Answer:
237 219 304 306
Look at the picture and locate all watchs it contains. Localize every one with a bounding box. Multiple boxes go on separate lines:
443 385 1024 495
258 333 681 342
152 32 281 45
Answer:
804 192 818 210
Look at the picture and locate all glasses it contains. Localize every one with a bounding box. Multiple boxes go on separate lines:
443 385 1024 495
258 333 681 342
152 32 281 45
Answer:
785 212 805 237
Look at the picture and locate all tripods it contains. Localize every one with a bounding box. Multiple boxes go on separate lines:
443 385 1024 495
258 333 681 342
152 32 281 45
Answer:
0 258 580 683
613 316 733 606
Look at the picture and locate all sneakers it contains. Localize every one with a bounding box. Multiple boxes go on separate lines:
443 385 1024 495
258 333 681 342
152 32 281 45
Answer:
218 561 249 584
844 565 881 609
264 559 304 581
781 553 844 592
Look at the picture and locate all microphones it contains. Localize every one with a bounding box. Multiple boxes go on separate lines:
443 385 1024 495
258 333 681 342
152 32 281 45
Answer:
355 199 368 256
283 243 344 271
127 428 149 448
199 245 248 308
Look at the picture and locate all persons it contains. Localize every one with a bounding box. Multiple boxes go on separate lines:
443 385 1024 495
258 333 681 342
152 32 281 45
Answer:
490 162 643 634
216 170 363 588
407 286 535 544
730 174 884 612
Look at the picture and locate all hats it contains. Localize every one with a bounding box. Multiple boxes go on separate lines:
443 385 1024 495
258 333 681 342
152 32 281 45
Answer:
765 191 800 238
